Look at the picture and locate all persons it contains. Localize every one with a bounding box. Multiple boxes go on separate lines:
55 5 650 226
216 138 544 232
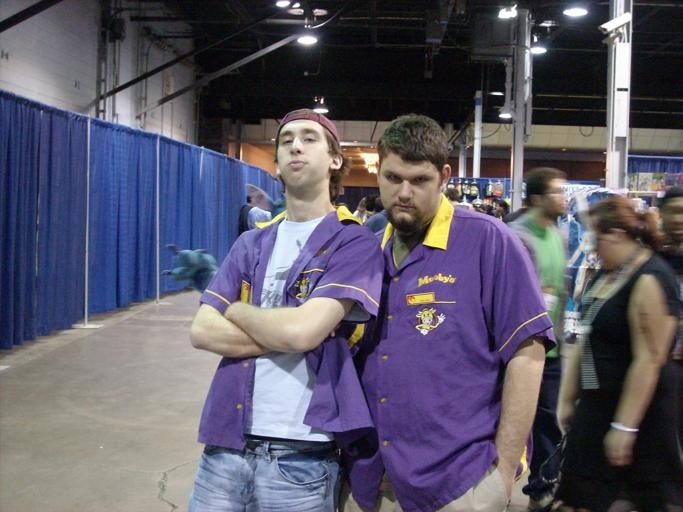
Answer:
507 165 573 511
337 111 558 511
184 107 386 511
554 193 683 511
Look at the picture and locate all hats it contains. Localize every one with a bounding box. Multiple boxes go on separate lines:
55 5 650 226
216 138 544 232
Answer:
275 107 341 149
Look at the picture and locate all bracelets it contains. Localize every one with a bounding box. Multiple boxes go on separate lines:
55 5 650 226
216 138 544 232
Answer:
611 422 642 434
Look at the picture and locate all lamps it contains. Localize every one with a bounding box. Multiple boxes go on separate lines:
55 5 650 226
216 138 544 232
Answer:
311 94 330 116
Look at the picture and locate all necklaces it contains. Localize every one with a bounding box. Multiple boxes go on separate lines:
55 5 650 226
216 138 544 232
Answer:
576 245 647 390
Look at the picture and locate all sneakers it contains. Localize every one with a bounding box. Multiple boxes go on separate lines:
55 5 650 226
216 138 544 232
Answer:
528 492 553 512
